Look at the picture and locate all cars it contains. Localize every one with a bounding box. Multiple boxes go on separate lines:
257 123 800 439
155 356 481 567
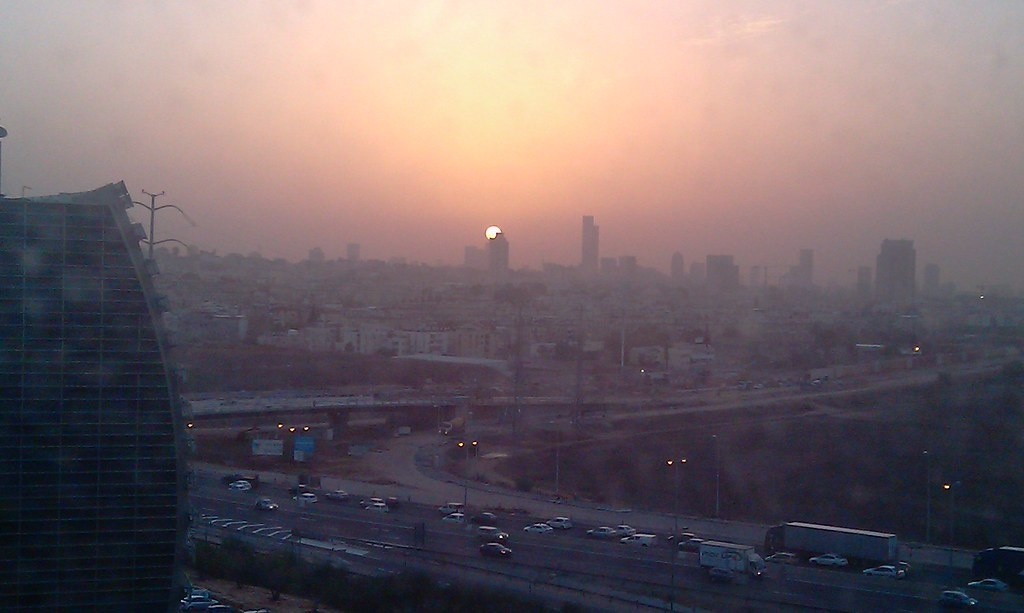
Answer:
863 563 906 579
290 485 315 496
292 492 318 505
667 532 698 547
968 578 1010 595
677 538 704 553
808 551 850 569
361 492 660 549
480 543 514 561
179 579 233 613
228 479 252 493
765 552 801 565
709 566 737 582
325 489 350 503
256 498 279 513
892 560 914 576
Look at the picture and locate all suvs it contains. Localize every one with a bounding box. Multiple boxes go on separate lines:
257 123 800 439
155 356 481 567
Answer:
939 590 979 611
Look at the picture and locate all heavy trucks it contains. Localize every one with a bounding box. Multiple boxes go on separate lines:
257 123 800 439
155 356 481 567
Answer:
765 522 898 564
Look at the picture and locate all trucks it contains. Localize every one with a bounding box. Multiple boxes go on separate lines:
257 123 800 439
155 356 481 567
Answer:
699 540 768 580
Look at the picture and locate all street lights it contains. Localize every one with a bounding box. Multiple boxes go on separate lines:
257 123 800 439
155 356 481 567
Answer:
127 187 194 271
